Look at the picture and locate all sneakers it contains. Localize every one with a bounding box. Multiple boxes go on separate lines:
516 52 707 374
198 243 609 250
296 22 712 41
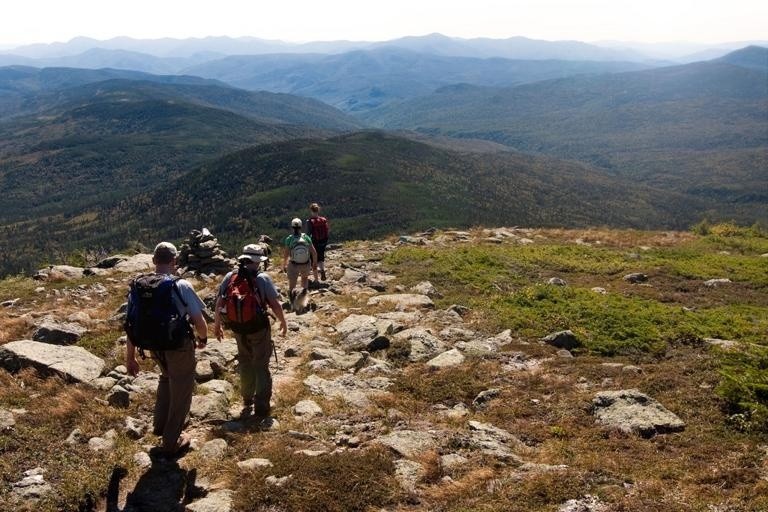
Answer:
177 436 190 450
153 423 163 436
244 394 274 414
313 281 319 288
321 270 325 280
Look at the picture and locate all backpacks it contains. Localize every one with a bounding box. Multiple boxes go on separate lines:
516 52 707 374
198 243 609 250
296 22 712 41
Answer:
123 275 196 360
221 269 276 323
306 216 329 241
288 233 310 263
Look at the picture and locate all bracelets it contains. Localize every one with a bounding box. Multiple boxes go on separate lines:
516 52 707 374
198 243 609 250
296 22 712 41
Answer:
196 336 208 345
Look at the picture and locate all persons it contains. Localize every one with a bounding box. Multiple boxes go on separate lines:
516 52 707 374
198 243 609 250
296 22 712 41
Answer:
282 217 318 290
214 243 288 417
305 203 328 284
126 240 208 456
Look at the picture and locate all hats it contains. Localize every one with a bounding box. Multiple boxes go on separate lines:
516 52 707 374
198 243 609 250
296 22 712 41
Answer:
291 218 302 228
153 242 181 259
237 244 269 263
